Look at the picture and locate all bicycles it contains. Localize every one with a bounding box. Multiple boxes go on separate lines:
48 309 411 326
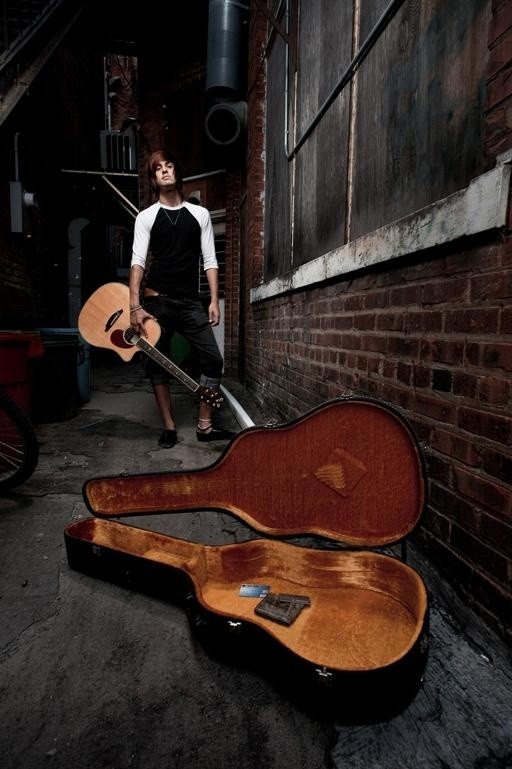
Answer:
0 391 40 492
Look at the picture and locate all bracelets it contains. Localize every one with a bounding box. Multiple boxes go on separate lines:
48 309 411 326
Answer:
129 305 142 313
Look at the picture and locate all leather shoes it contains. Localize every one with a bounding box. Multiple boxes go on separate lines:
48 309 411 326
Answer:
195 427 237 442
158 428 178 448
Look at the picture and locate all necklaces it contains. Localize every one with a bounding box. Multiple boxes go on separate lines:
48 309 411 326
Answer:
161 201 183 225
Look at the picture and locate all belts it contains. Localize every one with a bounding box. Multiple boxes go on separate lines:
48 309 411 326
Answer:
143 288 171 298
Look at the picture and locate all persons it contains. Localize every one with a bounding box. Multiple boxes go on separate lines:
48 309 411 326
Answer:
128 150 237 449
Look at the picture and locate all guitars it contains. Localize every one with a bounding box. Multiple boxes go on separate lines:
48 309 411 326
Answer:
78 283 224 408
